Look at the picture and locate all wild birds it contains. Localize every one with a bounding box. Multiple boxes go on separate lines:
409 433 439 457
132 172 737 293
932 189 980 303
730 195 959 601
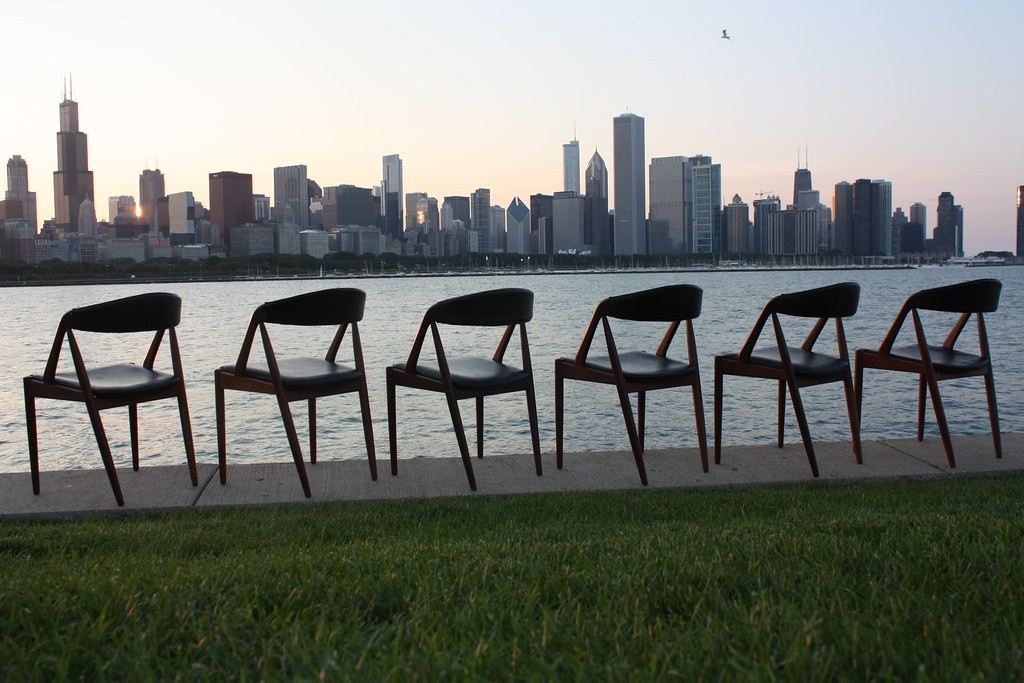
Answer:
720 29 731 41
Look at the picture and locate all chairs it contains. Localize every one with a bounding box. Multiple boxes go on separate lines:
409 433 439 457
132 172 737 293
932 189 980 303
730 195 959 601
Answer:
384 289 544 489
214 287 378 496
714 281 865 480
23 292 199 506
554 283 712 488
855 277 1006 471
